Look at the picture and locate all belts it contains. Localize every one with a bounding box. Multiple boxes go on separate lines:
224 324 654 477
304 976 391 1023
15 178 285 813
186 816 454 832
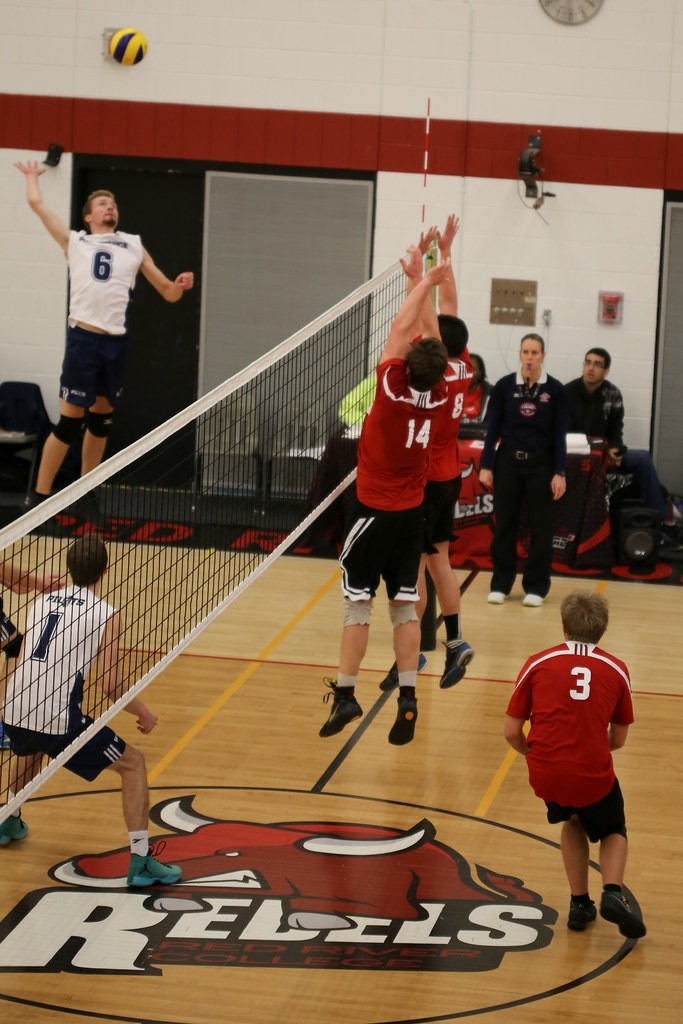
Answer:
499 444 547 460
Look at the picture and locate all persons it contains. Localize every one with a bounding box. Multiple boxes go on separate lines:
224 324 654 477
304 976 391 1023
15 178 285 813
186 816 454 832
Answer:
556 349 625 469
479 334 566 607
319 214 474 746
456 354 496 468
16 160 194 522
0 532 182 887
505 591 647 939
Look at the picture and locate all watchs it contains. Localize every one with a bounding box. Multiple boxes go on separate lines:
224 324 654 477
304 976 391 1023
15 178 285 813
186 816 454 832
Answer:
555 470 567 476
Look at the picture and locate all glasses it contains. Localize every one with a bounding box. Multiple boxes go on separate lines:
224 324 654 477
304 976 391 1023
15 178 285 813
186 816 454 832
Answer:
583 360 606 368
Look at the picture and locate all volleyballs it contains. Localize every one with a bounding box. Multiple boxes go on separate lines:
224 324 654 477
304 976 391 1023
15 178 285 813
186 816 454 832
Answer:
109 26 149 66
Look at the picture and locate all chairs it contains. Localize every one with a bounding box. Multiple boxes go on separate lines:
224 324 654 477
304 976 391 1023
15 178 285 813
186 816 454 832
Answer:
192 391 262 516
0 381 55 506
260 397 329 515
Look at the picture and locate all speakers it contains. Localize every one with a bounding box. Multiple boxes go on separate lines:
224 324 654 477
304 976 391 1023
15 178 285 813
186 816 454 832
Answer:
619 509 661 568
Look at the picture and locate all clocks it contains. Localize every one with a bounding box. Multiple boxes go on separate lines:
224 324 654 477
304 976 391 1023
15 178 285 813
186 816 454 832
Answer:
539 0 603 25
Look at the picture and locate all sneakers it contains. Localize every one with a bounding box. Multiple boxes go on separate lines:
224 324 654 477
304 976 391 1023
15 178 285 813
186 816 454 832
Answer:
319 677 363 737
388 697 418 745
568 900 597 929
0 721 10 748
0 812 28 843
439 640 474 689
63 491 111 527
127 841 181 886
600 891 646 938
522 593 546 606
19 489 66 536
379 653 428 690
487 591 510 603
